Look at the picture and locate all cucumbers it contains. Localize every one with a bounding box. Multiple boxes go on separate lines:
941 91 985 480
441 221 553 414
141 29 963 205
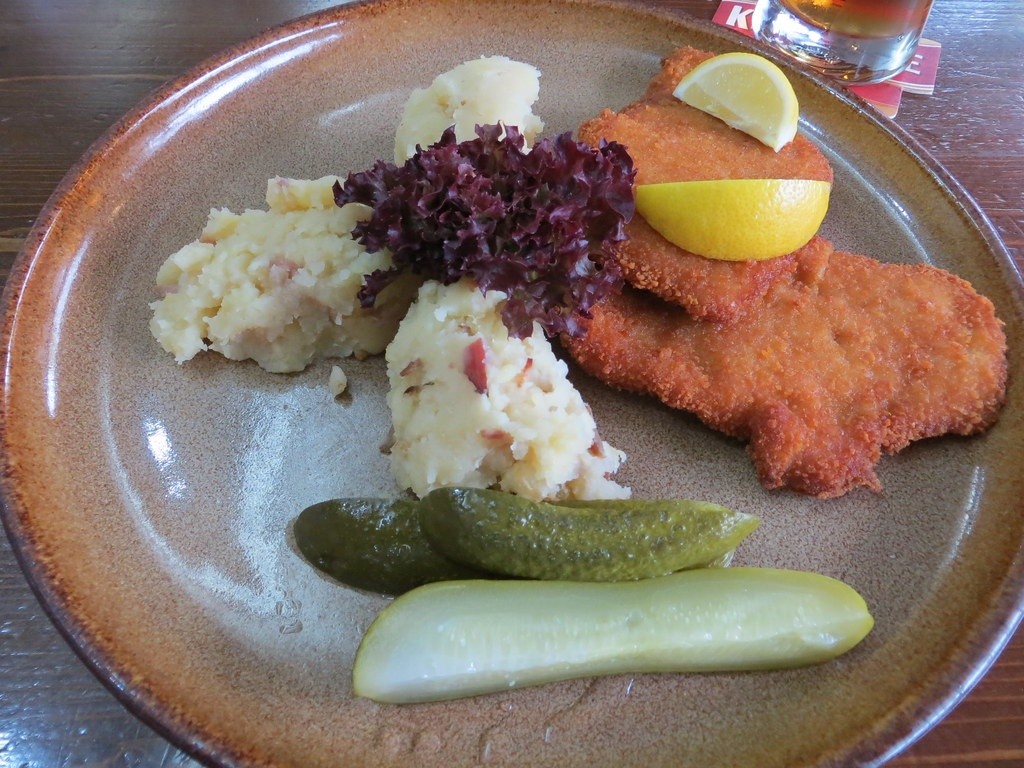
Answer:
291 484 875 702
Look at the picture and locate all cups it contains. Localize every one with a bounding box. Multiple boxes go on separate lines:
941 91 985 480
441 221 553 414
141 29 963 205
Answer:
749 0 935 85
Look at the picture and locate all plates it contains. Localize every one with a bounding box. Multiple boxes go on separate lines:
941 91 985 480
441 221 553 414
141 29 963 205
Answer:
1 0 1024 768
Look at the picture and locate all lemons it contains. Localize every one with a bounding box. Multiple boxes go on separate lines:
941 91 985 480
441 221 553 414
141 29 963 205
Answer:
630 178 832 262
671 50 799 154
